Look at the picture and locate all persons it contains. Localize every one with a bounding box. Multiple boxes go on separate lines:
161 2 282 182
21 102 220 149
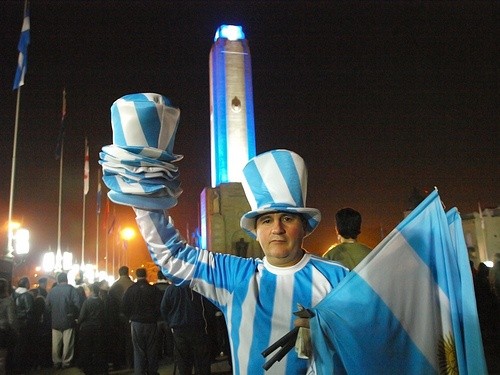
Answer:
0 267 230 375
477 260 489 315
322 208 372 272
470 260 478 273
99 92 352 375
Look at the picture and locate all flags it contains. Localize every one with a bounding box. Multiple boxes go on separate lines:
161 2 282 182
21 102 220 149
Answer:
62 88 66 120
311 190 487 375
83 140 90 196
12 0 30 90
97 173 126 250
478 202 485 230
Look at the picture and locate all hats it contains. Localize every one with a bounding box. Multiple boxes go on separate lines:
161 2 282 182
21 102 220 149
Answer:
240 149 321 239
98 92 183 209
